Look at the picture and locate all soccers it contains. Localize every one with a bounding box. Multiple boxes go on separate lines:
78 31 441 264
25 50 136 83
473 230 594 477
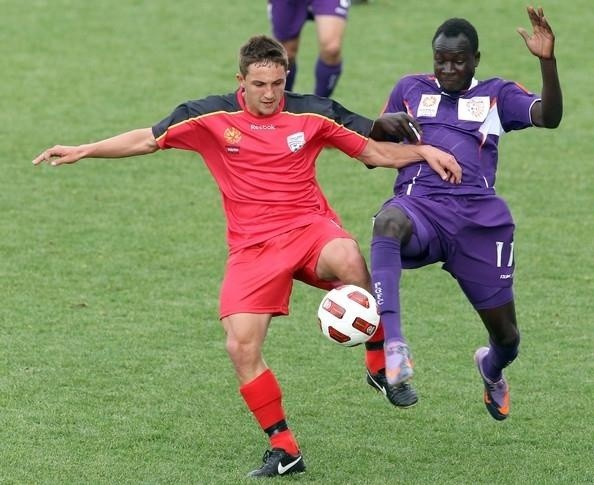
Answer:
317 284 380 347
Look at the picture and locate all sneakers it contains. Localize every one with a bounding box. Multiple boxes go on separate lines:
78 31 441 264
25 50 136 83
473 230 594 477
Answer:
243 446 306 480
363 366 420 410
381 340 414 389
472 344 512 422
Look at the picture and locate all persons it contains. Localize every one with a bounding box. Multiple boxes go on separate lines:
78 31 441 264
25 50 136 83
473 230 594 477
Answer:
30 32 466 478
266 0 353 98
369 2 566 422
